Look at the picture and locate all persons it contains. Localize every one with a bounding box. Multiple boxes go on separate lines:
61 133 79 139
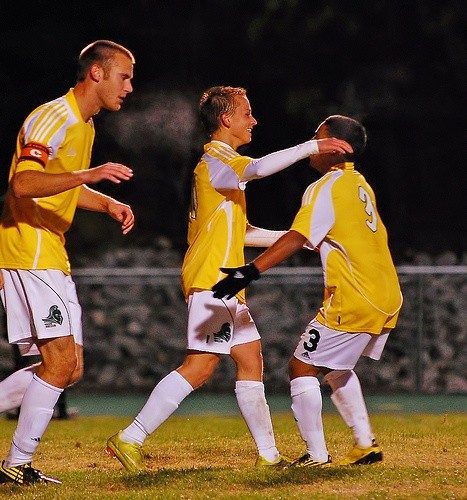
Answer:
0 238 467 396
212 115 405 472
0 39 136 494
106 85 353 476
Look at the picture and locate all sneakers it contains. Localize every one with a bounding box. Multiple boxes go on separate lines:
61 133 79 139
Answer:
255 454 296 471
106 430 146 473
282 453 332 471
0 460 62 492
336 438 383 466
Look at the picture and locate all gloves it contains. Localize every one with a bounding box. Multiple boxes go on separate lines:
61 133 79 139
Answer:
211 262 261 300
